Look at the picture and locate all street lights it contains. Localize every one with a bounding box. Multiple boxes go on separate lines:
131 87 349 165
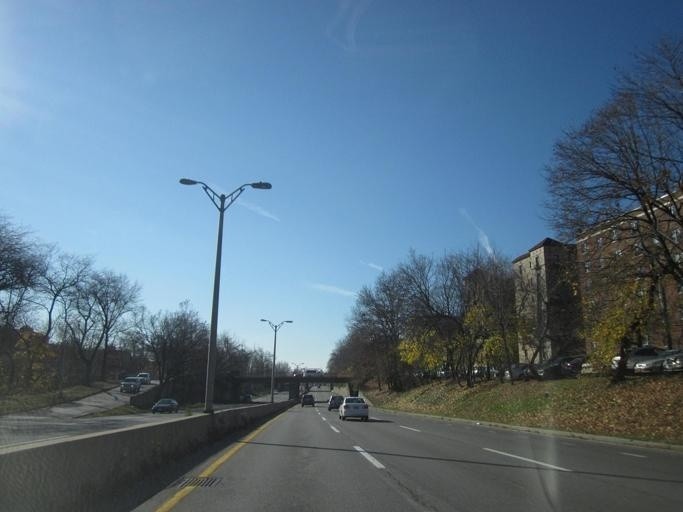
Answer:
259 317 293 404
178 177 271 414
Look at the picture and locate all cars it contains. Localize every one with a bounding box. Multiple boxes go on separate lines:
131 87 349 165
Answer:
609 345 682 374
411 355 591 381
338 396 368 421
300 394 314 407
119 376 142 393
135 373 151 384
150 398 178 414
327 395 343 411
